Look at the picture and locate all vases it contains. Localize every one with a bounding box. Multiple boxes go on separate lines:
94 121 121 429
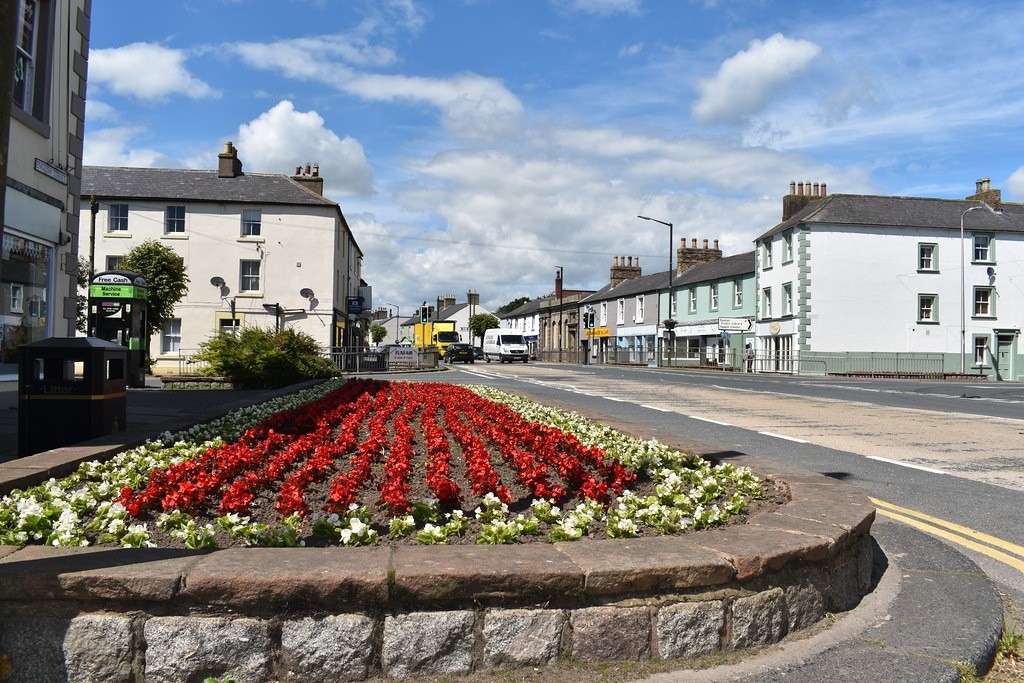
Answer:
665 324 675 329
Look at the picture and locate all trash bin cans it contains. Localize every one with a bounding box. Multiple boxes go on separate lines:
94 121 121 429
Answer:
18 338 128 459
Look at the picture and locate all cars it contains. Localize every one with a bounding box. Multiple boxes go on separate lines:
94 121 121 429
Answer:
363 341 413 357
443 343 474 364
473 347 484 360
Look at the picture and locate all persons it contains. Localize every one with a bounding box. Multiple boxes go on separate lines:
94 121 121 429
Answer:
742 344 754 373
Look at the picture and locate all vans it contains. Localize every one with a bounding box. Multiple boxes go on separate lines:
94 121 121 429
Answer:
482 328 529 364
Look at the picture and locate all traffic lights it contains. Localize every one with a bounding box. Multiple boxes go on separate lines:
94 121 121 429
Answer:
420 306 428 323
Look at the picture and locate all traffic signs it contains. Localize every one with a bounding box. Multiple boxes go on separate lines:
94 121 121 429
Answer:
718 317 753 331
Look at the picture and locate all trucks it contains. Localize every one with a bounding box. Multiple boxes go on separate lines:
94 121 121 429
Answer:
413 320 462 360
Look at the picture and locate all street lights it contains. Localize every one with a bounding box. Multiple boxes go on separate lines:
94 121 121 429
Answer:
961 206 984 374
386 302 399 344
555 265 564 361
637 215 673 367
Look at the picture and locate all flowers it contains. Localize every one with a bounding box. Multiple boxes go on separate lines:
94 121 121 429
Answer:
662 319 678 325
